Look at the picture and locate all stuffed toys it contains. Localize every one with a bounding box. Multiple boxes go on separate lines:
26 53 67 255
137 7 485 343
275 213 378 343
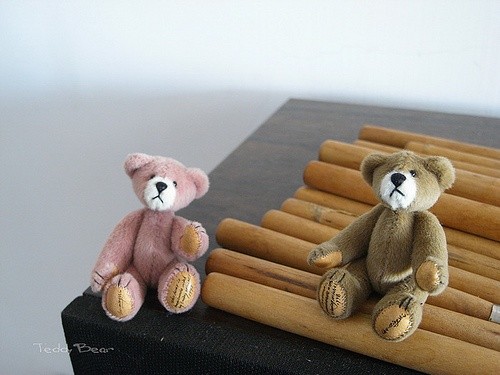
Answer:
90 151 209 322
308 149 456 344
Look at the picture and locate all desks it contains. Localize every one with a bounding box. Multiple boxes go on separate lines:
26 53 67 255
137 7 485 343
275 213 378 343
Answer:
61 99 500 374
